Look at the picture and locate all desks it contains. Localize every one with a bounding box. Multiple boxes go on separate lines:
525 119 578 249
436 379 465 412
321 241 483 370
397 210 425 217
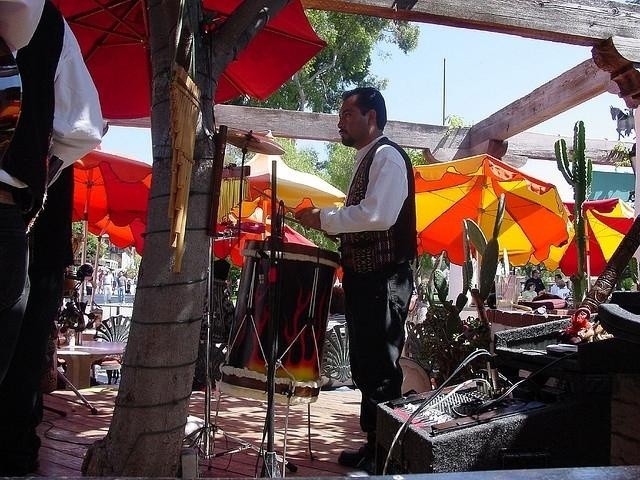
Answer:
56 346 124 390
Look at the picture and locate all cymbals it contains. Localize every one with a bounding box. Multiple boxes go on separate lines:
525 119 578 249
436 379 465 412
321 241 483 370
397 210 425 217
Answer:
216 125 285 156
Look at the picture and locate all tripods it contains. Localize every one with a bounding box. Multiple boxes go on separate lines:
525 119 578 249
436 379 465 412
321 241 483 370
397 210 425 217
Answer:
183 238 298 472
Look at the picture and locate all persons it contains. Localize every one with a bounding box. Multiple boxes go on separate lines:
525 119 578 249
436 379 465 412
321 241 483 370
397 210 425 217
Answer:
555 279 571 299
117 271 128 304
521 282 539 303
0 165 77 480
523 270 547 295
110 272 117 295
550 273 568 293
293 83 420 477
97 269 105 295
0 0 106 382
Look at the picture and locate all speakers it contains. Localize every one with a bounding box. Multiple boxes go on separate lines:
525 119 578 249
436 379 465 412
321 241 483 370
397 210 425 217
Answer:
492 321 612 467
376 385 550 474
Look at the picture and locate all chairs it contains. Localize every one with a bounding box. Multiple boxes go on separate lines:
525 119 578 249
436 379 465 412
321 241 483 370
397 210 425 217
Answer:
92 315 132 385
321 321 359 390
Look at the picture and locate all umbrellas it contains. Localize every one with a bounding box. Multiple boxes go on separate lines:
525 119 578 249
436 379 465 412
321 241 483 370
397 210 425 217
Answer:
47 0 331 123
70 150 155 301
408 153 570 289
211 129 349 233
526 194 640 291
212 206 321 271
86 214 147 314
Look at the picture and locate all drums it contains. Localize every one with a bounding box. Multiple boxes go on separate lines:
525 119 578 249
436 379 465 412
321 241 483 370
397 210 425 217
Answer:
212 238 342 405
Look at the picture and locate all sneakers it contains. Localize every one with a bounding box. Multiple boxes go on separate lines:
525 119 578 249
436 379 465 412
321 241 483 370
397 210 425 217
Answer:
337 430 377 472
